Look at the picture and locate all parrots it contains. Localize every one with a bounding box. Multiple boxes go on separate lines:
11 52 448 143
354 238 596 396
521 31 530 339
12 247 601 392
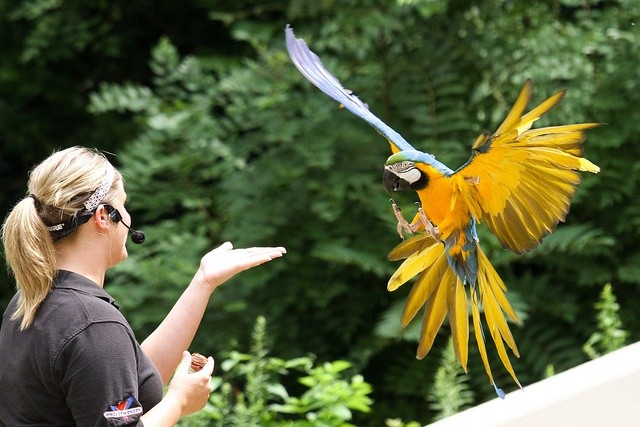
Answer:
283 22 609 400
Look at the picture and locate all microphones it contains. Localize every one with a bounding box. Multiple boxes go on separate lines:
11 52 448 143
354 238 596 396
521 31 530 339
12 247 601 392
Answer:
121 219 145 244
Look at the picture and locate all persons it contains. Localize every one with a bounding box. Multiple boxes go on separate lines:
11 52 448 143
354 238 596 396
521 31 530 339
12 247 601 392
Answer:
0 142 288 426
